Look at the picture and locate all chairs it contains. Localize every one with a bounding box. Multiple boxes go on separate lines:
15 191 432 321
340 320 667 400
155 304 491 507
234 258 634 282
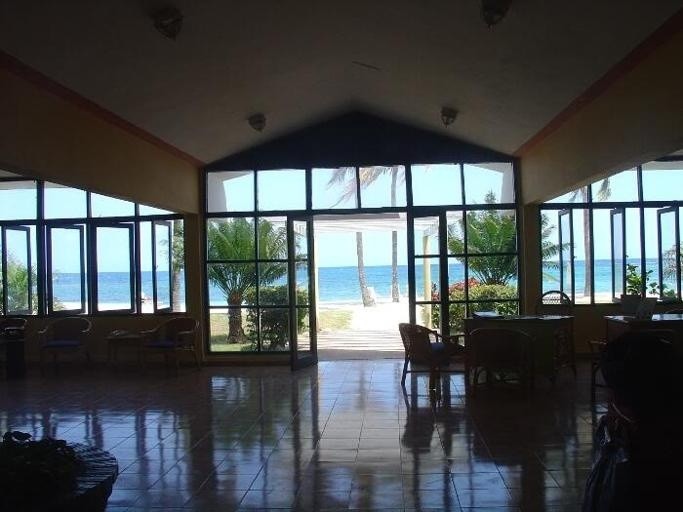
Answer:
399 290 576 398
0 316 202 378
585 327 683 471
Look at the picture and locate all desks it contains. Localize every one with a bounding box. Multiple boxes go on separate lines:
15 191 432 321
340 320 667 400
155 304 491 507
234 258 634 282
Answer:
603 314 683 345
0 436 118 511
